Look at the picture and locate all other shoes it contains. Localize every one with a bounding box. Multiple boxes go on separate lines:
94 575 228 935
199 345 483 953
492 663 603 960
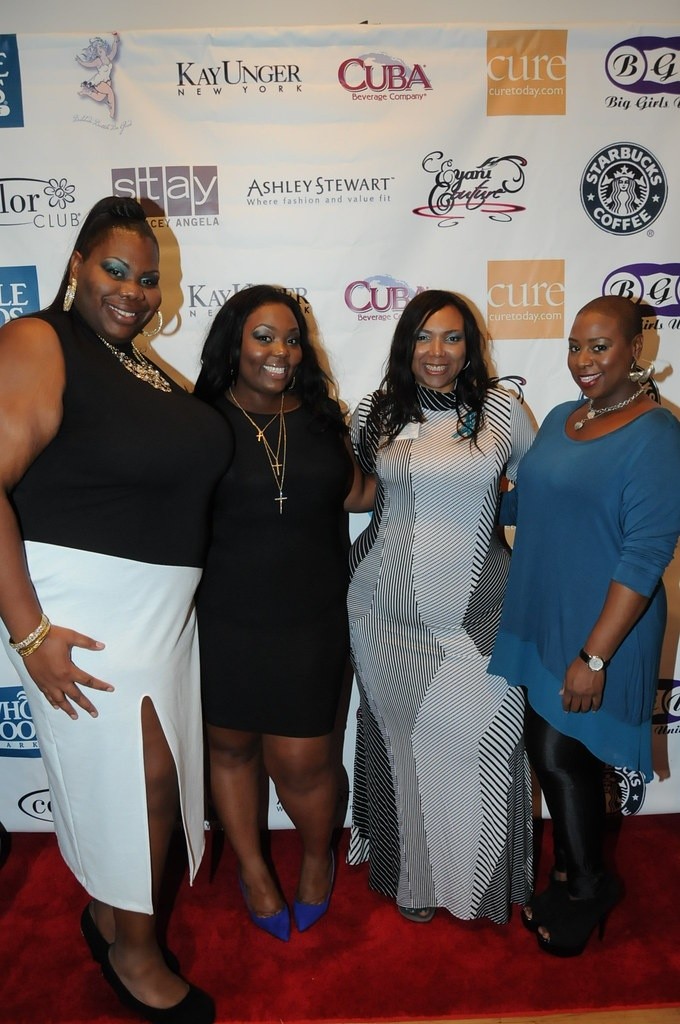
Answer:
239 871 290 941
398 905 436 922
293 848 335 931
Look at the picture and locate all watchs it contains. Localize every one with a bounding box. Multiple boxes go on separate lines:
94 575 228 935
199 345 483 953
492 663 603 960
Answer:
578 647 608 672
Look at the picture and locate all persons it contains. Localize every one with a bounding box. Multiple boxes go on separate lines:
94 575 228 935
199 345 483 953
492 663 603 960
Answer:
459 295 680 958
0 198 233 1024
189 285 378 945
348 291 539 926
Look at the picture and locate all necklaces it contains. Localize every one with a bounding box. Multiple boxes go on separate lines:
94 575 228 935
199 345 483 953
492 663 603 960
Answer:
98 332 171 393
230 389 289 516
574 381 645 430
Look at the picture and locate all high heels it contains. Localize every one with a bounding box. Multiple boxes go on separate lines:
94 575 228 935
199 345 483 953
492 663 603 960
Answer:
537 872 620 957
100 943 216 1024
81 901 180 982
521 865 571 931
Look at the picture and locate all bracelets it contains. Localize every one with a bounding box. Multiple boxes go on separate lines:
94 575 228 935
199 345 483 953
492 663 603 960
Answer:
9 613 50 658
